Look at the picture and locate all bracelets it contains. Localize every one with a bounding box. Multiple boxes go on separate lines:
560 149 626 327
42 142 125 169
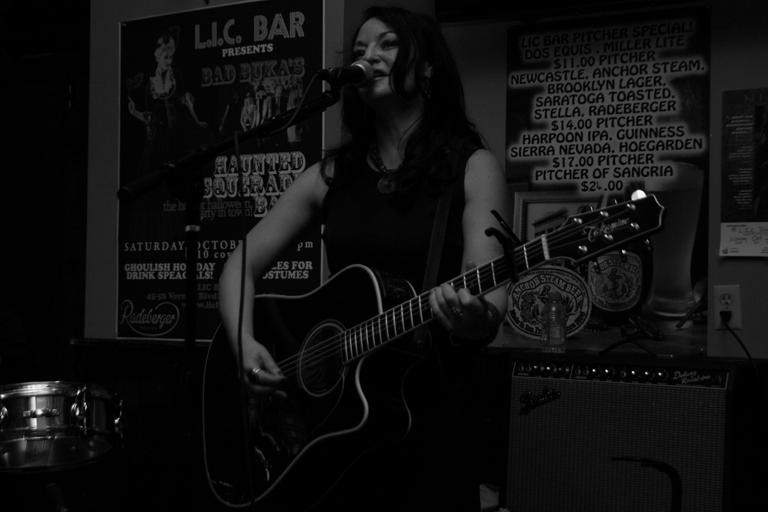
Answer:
484 300 501 328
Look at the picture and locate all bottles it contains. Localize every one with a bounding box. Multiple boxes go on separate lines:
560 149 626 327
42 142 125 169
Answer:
540 291 570 358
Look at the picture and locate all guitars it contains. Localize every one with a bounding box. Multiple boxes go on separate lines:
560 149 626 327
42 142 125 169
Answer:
198 190 669 507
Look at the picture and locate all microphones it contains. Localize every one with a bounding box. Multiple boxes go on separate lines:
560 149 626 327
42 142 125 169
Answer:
319 56 375 108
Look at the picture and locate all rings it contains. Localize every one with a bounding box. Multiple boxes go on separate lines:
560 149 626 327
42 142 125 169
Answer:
248 366 261 379
451 306 466 318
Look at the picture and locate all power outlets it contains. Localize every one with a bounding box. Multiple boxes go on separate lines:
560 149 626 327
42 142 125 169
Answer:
713 281 744 333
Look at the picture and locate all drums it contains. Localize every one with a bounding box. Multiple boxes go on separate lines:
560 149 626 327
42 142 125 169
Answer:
0 381 128 475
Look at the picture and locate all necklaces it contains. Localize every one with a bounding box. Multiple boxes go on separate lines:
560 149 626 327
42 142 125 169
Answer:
367 142 406 194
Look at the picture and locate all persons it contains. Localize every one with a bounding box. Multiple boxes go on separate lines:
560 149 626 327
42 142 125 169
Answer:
125 24 210 133
217 5 510 510
240 77 305 133
